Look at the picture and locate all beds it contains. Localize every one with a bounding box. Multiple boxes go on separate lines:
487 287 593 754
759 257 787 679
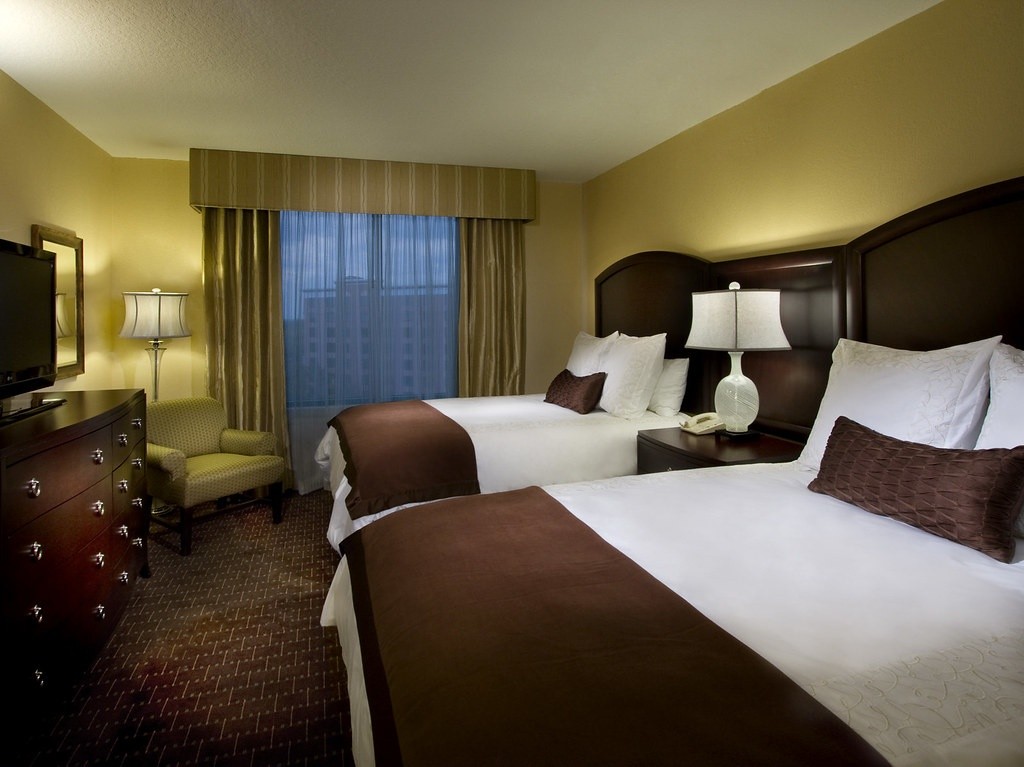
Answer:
318 461 1024 767
313 393 695 558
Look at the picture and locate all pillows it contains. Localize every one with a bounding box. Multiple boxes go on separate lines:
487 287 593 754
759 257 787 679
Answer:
593 333 668 421
566 331 619 377
796 334 1003 475
808 417 1024 564
973 343 1024 539
647 358 690 417
544 369 605 414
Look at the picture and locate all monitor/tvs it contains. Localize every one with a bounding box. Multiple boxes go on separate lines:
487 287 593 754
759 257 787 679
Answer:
0 239 67 425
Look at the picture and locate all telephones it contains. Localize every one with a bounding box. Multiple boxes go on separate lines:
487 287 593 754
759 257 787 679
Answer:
680 411 725 435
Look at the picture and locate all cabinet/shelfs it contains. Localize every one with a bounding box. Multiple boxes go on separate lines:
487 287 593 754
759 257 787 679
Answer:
0 389 151 738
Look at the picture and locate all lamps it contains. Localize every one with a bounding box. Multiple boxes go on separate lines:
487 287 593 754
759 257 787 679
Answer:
55 292 73 339
118 289 192 402
684 281 792 435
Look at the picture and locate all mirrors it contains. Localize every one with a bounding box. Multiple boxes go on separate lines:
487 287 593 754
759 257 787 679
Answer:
30 224 85 379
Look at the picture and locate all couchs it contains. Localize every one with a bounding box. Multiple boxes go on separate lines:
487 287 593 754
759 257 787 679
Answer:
145 398 285 554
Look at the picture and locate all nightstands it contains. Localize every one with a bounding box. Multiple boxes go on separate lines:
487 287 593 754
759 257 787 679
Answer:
637 427 804 475
